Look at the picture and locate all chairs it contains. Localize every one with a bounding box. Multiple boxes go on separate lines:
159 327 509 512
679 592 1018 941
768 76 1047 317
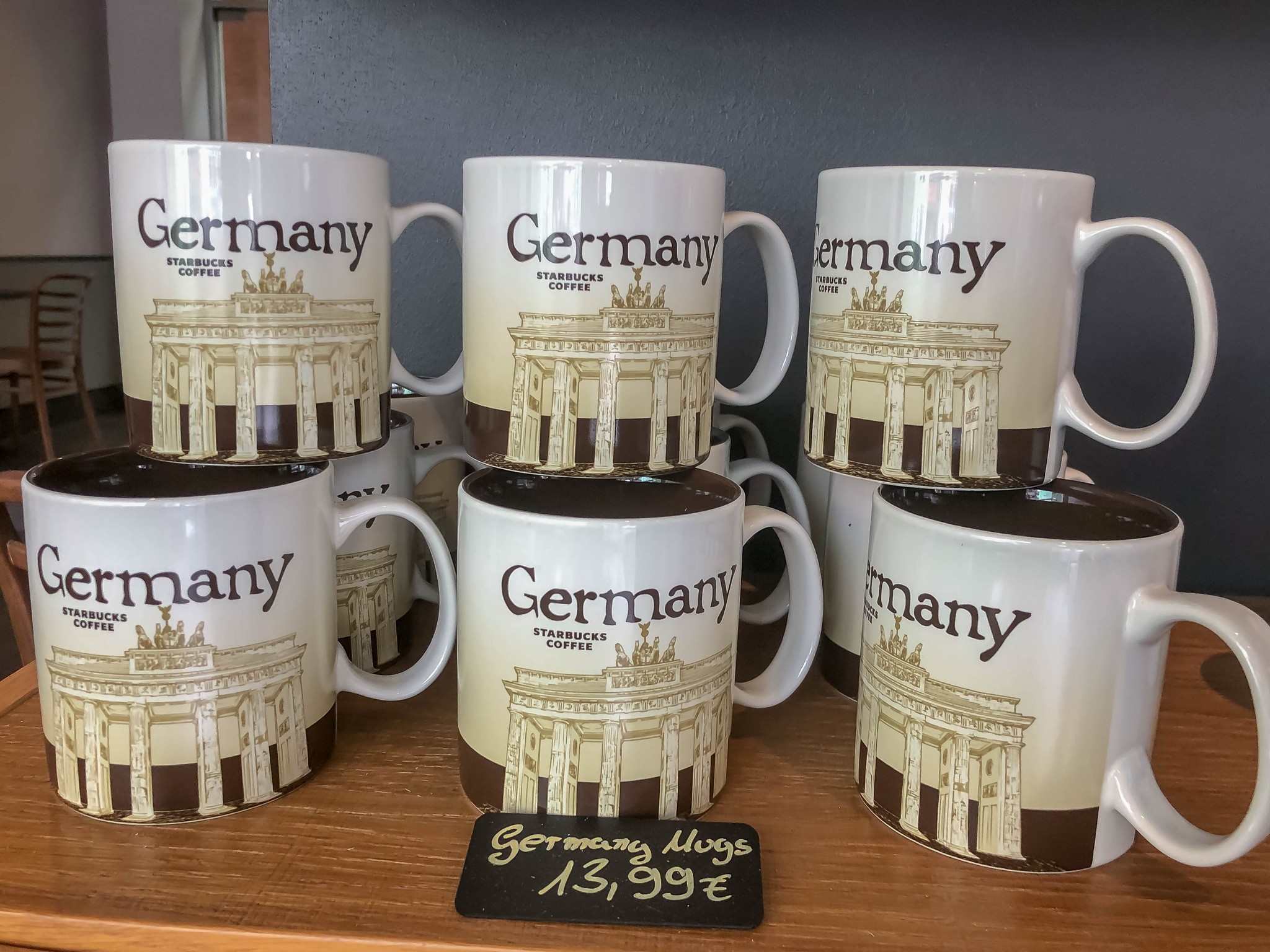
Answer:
0 274 106 666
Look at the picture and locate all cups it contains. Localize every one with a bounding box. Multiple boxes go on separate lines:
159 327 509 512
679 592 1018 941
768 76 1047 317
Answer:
459 155 801 482
104 137 463 469
802 166 1218 490
16 384 1270 877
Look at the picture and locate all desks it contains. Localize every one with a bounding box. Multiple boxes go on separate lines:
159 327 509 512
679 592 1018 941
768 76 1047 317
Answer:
0 595 1270 952
